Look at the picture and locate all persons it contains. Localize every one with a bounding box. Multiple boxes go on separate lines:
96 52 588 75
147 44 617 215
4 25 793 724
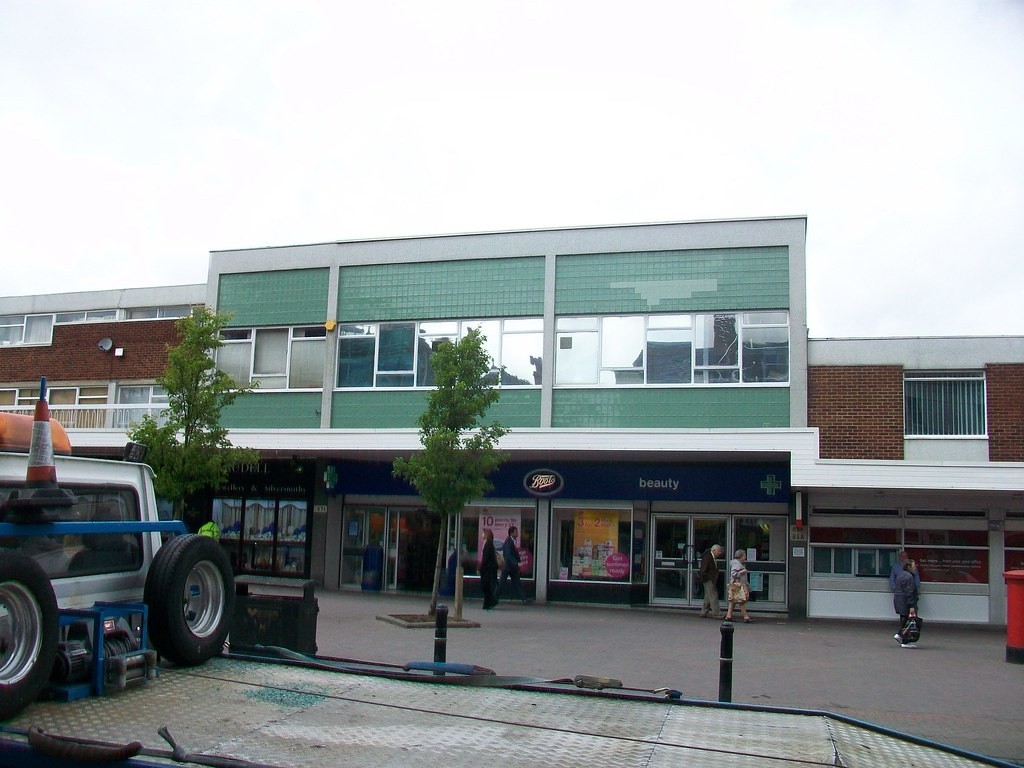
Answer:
478 528 499 610
725 550 757 623
495 526 536 605
892 558 920 649
699 545 728 619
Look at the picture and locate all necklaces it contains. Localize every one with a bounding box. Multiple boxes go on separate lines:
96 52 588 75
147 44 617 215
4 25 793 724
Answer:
889 550 921 632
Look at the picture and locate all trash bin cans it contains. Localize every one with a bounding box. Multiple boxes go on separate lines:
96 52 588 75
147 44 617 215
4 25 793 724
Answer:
1001 569 1024 662
232 575 321 658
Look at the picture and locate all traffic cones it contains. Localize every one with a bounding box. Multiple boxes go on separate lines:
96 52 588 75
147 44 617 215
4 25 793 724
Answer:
25 401 57 482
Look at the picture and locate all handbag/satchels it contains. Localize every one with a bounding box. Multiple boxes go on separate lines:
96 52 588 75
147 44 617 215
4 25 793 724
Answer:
495 552 503 569
901 614 923 642
727 572 749 604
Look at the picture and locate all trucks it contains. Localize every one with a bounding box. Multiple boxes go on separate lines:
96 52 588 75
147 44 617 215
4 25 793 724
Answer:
0 378 1024 768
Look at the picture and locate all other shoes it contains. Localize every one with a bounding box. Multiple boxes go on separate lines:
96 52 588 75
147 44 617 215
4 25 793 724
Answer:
726 617 739 621
483 600 498 609
522 596 536 604
744 617 756 623
701 613 724 619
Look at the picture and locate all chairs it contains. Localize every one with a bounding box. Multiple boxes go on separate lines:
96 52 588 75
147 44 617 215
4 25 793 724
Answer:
64 515 138 576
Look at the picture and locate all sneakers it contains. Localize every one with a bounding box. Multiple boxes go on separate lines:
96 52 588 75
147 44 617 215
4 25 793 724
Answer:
893 634 902 643
901 644 917 648
